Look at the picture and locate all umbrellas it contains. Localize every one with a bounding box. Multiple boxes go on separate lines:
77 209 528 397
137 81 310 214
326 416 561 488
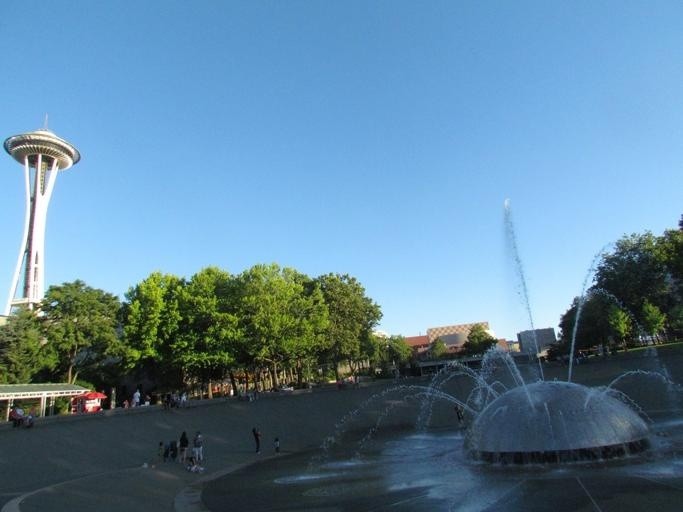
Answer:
76 391 108 400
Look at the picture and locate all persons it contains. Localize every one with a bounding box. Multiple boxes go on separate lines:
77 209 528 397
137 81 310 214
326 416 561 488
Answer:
133 388 141 407
123 399 128 408
251 427 262 456
238 383 262 403
143 394 151 407
337 372 361 392
156 429 207 474
8 404 36 430
161 389 188 412
273 437 280 456
454 404 463 423
